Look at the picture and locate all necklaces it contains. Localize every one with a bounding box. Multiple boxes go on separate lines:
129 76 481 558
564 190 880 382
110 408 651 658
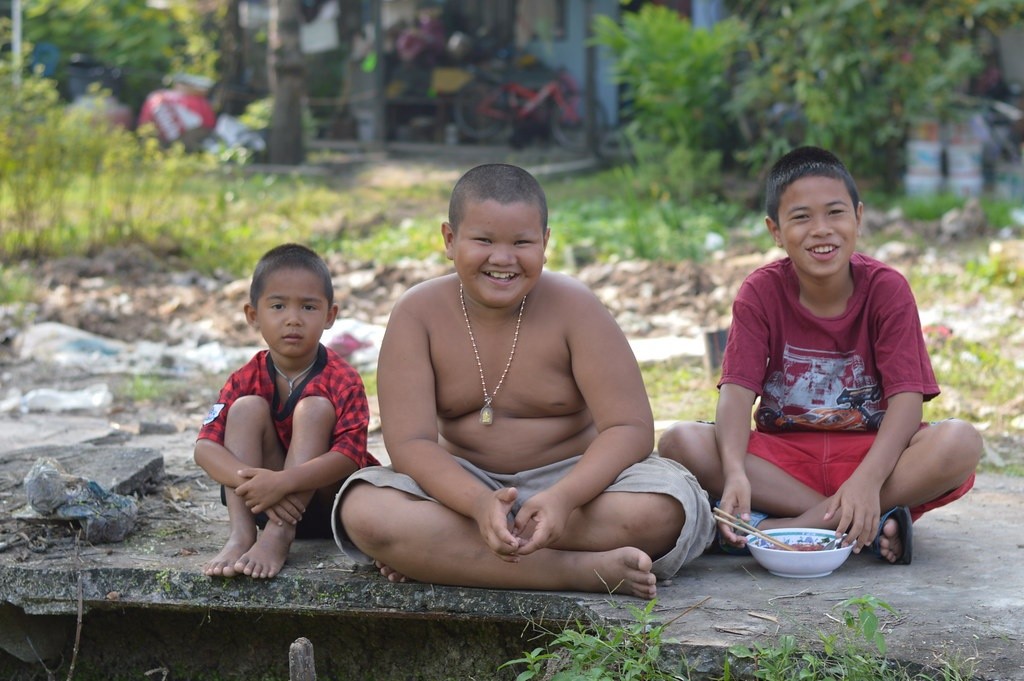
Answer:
460 283 526 425
273 359 316 397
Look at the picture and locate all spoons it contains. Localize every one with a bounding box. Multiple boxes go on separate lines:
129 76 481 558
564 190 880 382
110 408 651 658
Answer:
824 533 847 550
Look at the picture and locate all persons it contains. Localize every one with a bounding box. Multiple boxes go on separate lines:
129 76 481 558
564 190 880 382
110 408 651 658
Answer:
658 147 985 566
331 162 716 600
194 244 381 578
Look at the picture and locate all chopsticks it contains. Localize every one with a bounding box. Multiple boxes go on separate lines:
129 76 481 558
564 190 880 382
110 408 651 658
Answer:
713 507 793 551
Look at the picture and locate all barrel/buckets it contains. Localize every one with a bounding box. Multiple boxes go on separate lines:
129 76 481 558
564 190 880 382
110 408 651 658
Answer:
904 140 983 198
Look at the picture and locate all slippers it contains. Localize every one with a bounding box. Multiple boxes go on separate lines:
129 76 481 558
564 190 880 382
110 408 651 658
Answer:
865 504 912 566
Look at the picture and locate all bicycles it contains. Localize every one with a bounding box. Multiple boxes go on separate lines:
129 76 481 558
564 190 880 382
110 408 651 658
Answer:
460 42 609 153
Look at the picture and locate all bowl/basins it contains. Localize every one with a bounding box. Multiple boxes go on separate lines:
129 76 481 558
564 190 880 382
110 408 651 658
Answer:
744 527 857 579
